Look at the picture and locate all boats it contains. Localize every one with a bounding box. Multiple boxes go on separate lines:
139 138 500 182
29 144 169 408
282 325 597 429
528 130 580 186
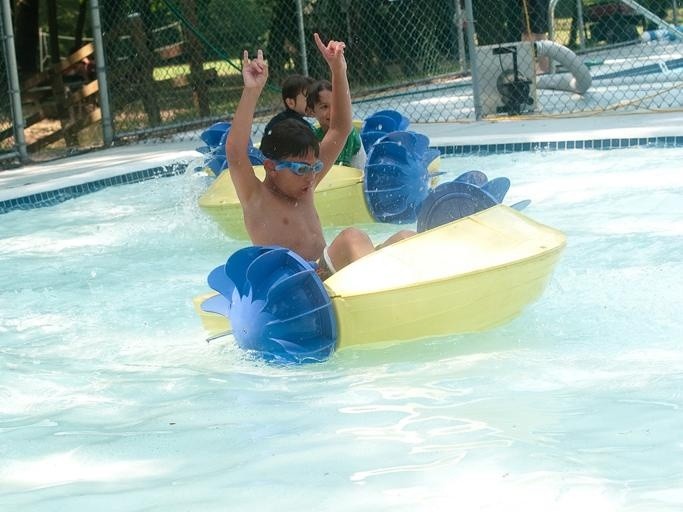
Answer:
195 171 566 366
196 110 445 241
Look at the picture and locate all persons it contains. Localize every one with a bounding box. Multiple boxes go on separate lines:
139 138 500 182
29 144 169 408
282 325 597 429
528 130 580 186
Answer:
305 80 368 172
567 0 668 52
89 0 155 84
282 75 315 117
521 0 559 75
226 32 417 283
255 0 327 84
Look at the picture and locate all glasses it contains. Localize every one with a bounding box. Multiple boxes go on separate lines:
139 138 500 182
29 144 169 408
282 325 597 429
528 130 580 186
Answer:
271 157 323 175
